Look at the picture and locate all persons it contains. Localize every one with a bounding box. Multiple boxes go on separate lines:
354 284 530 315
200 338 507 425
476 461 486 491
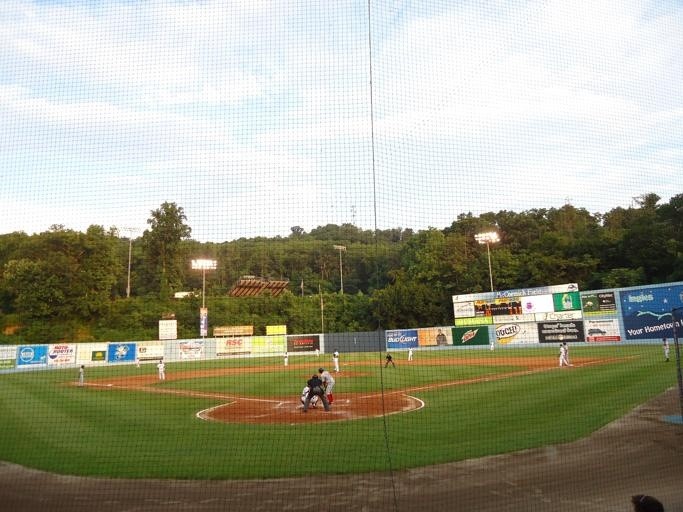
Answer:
436 329 448 346
491 341 494 352
332 348 340 374
562 342 569 364
408 346 413 361
662 338 670 362
137 357 140 367
559 344 568 367
157 361 165 380
284 352 288 366
383 353 397 368
298 367 335 411
78 365 85 386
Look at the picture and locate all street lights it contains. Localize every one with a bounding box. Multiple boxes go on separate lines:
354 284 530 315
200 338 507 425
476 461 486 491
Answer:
192 259 218 339
474 232 499 292
118 225 142 299
332 244 347 296
